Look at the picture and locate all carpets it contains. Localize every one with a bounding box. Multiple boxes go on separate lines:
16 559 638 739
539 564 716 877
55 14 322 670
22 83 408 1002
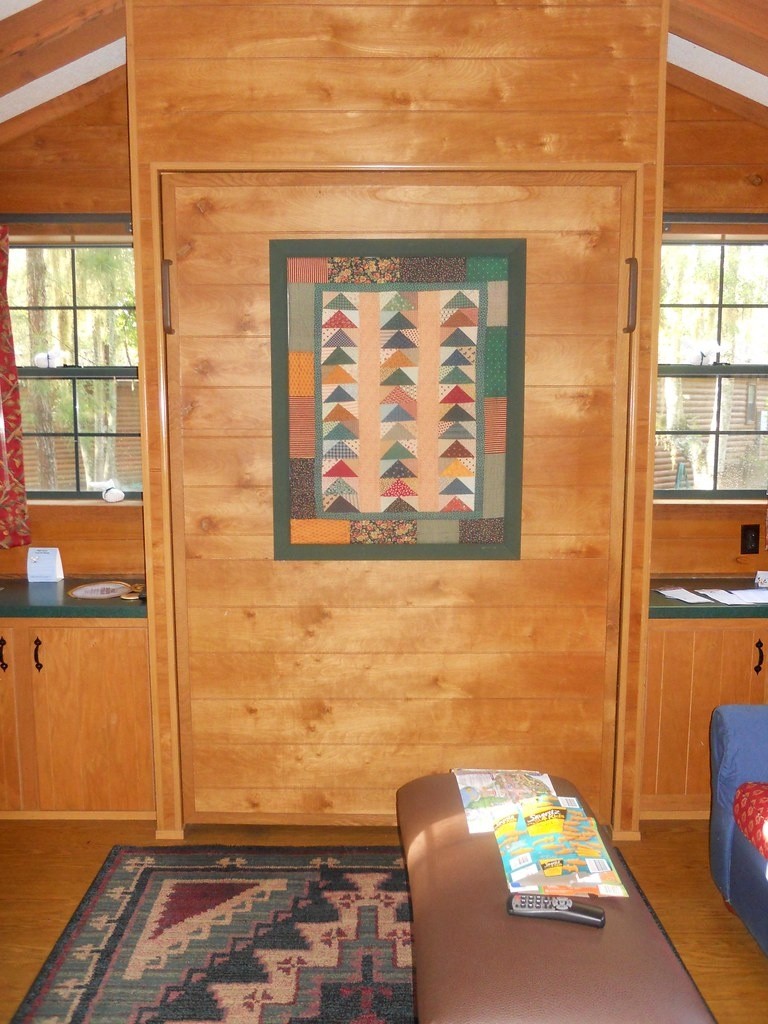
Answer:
9 843 416 1024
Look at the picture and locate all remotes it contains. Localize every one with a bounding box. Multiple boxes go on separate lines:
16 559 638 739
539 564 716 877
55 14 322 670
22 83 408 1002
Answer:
507 893 606 929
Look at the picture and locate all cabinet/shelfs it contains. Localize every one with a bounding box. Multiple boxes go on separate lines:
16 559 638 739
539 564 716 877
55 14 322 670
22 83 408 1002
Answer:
642 608 768 823
0 617 158 822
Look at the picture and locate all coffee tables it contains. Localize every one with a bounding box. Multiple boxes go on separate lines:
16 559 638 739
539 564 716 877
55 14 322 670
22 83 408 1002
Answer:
396 769 718 1024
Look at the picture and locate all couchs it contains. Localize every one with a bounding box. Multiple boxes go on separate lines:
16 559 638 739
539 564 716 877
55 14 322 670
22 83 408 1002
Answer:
706 704 768 955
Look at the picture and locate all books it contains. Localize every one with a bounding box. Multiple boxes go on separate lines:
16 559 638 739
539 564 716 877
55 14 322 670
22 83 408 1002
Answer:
450 763 562 835
493 796 630 899
